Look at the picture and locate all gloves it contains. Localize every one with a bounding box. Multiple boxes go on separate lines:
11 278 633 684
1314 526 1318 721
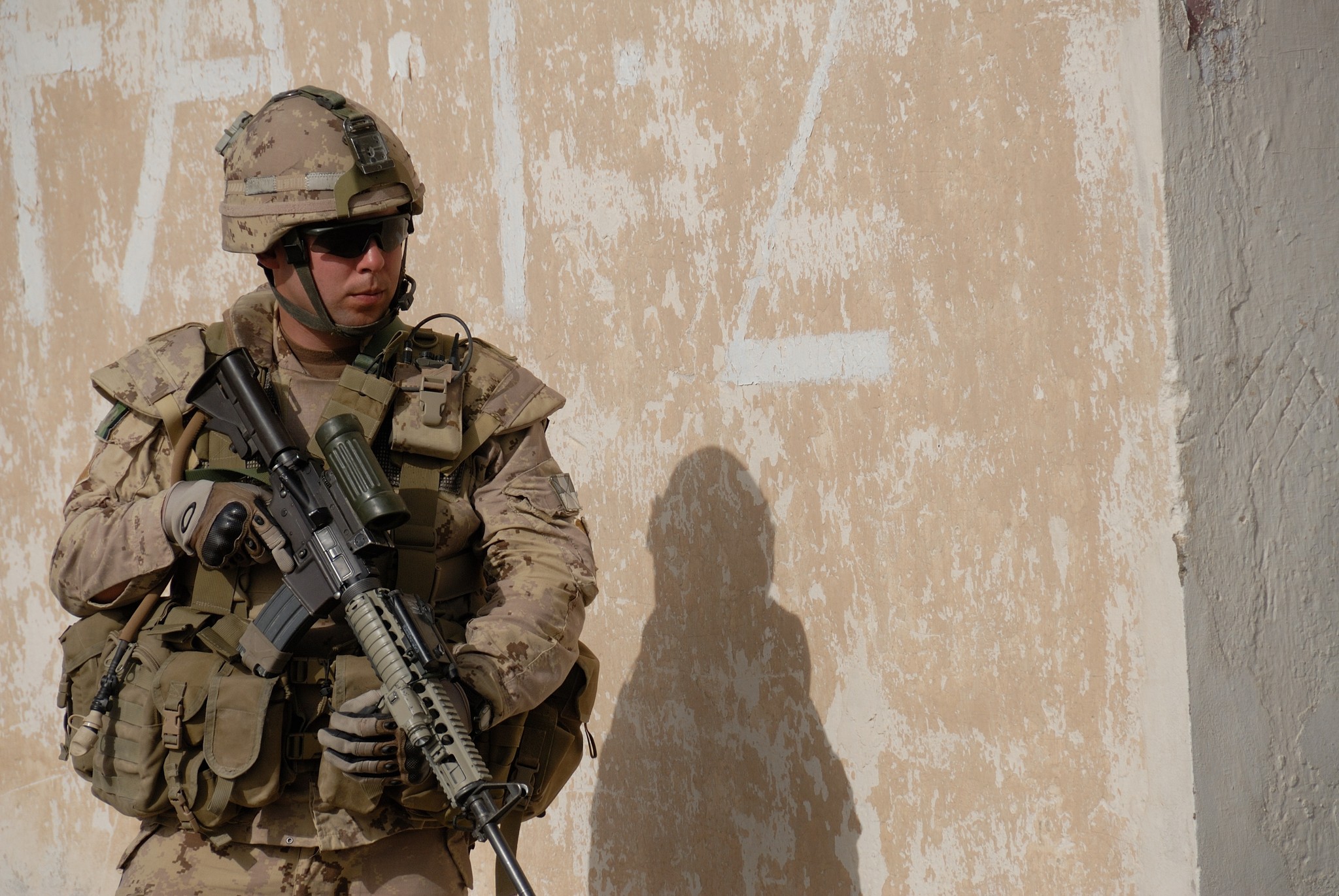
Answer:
317 678 492 785
161 479 296 572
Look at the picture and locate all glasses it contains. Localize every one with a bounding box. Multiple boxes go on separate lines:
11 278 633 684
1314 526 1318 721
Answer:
298 212 410 259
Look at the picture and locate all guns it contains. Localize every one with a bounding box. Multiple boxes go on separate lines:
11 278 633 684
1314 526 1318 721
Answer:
184 346 540 895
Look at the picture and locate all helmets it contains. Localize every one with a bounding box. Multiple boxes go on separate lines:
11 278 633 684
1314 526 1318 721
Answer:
221 87 426 254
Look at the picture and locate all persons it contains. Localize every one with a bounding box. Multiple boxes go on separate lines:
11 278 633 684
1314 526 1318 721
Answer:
50 104 597 896
